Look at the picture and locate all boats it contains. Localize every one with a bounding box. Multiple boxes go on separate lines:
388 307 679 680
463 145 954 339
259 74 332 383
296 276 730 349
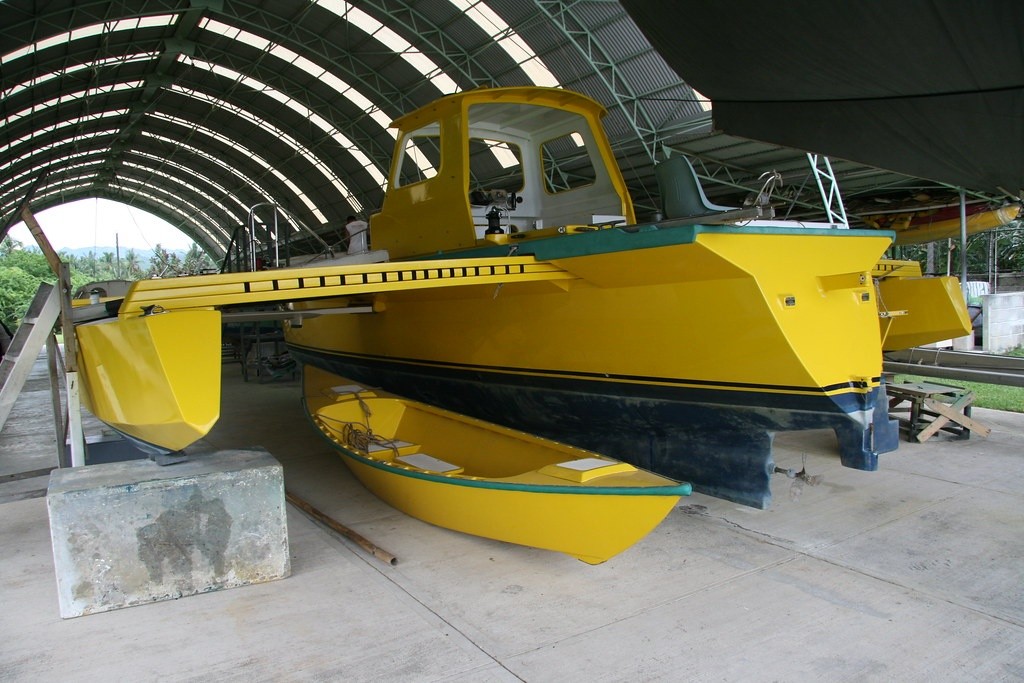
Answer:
74 86 973 510
302 363 691 566
